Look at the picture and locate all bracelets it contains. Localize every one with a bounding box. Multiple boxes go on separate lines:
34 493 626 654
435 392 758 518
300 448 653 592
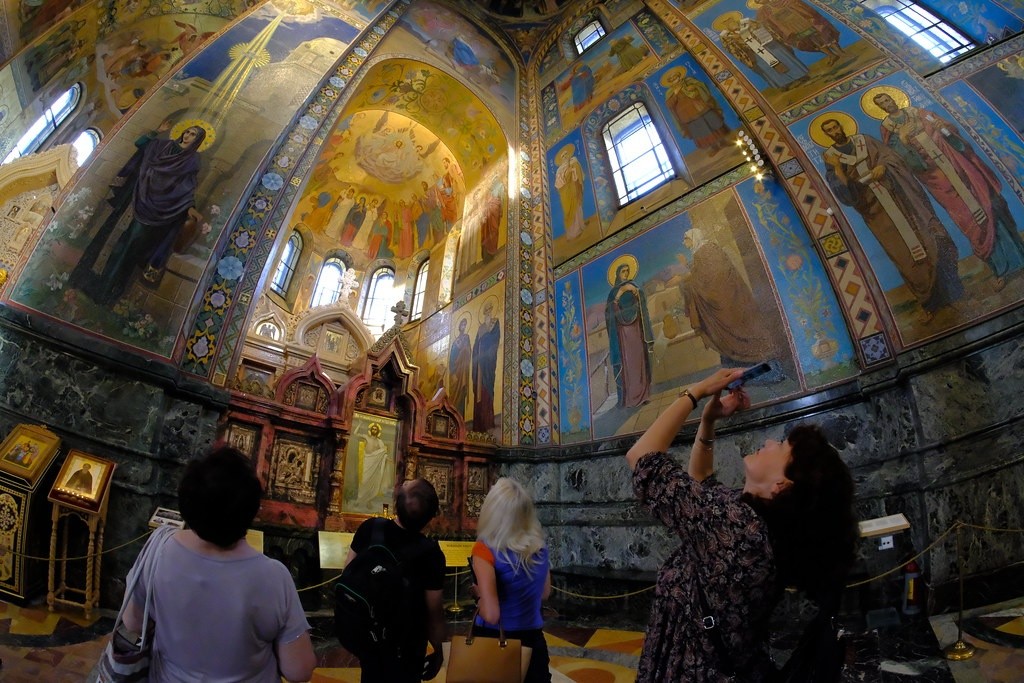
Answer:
678 389 698 410
696 435 717 446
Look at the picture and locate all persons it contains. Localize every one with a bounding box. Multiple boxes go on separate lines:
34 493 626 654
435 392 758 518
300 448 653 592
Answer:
624 367 861 683
120 444 318 683
470 477 552 683
342 476 446 683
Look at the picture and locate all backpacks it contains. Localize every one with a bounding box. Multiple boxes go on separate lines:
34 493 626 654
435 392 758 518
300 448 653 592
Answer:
333 526 434 665
695 496 844 683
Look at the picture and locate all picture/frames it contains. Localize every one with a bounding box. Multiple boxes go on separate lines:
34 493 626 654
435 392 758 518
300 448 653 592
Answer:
0 427 55 480
53 451 113 510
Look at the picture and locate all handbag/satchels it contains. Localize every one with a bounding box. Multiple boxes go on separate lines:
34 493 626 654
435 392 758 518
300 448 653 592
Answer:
445 606 520 683
88 525 175 683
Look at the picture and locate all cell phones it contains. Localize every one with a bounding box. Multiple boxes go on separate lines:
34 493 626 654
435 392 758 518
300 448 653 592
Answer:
725 362 772 391
